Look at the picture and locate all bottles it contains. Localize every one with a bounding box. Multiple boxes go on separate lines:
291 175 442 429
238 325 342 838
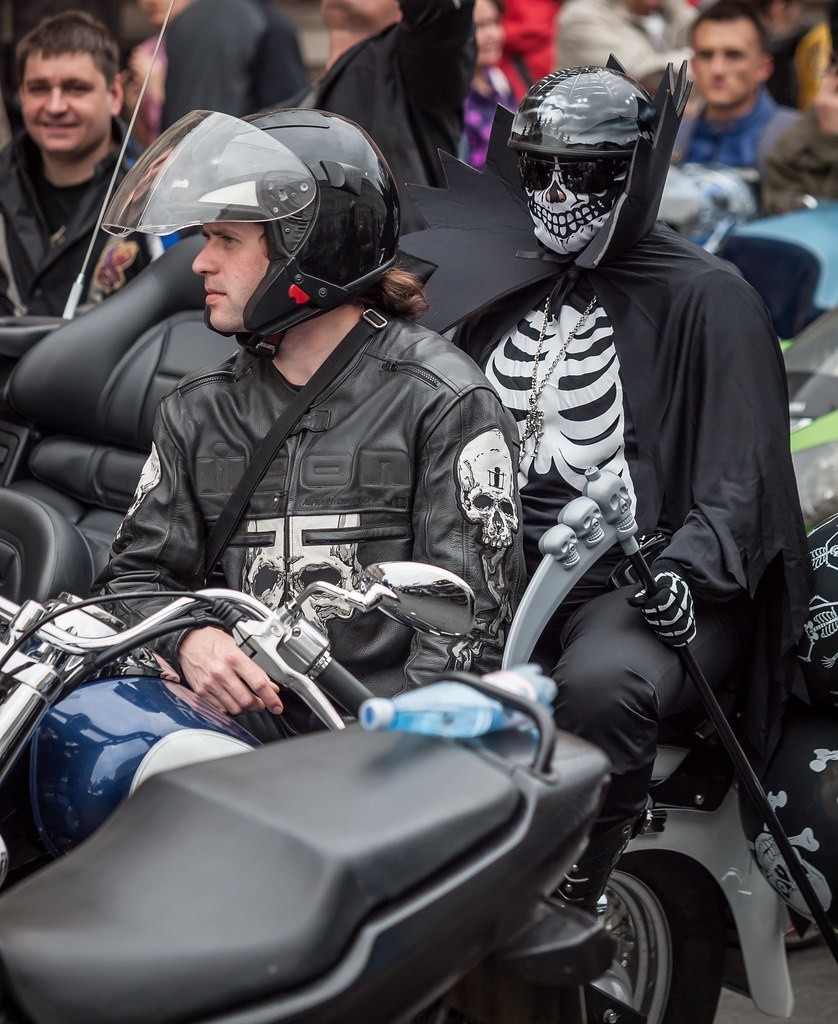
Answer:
362 664 559 741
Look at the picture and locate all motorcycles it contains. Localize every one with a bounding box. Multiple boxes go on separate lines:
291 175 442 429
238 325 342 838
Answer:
0 0 838 1023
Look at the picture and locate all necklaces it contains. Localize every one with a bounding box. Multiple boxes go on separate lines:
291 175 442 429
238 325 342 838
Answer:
510 290 597 462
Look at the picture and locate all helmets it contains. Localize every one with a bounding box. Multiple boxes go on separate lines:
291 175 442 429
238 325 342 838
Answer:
167 107 405 361
509 65 658 154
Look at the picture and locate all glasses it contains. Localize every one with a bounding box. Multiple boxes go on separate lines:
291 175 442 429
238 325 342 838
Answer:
506 149 629 194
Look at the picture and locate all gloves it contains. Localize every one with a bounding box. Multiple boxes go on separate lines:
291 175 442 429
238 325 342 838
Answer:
627 559 700 650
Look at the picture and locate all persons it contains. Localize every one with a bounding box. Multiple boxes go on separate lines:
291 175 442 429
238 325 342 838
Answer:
0 11 157 323
446 2 838 217
275 0 478 303
128 0 314 136
448 64 838 958
89 104 530 743
